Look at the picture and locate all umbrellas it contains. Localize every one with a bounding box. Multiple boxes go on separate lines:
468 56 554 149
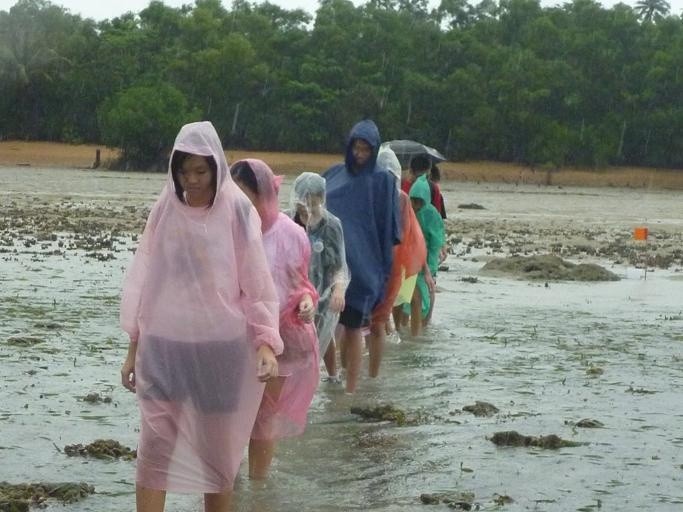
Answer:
382 138 448 170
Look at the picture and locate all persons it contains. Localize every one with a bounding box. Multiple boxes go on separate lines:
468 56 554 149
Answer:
367 140 426 382
282 171 352 369
119 120 284 511
382 159 447 341
321 118 403 400
229 157 321 481
